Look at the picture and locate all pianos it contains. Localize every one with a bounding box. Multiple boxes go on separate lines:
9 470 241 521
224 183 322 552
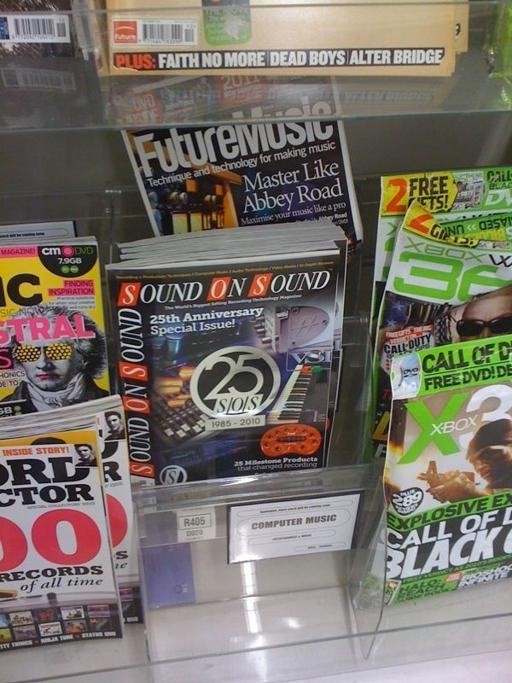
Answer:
260 354 312 426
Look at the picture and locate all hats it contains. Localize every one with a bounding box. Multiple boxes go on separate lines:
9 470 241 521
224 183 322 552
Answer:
467 418 511 459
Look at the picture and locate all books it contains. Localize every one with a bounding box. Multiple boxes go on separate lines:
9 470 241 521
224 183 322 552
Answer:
105 219 347 513
0 221 143 651
0 0 469 77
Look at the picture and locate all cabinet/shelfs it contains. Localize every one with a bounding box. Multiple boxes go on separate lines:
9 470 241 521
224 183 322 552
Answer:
0 64 510 683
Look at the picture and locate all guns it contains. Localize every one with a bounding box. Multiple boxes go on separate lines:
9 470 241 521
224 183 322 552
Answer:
416 459 475 488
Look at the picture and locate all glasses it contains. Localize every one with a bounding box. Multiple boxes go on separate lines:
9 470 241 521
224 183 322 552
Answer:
12 343 91 362
457 317 512 336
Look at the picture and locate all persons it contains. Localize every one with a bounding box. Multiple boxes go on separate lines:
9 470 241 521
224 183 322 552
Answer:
454 284 512 341
419 419 512 506
